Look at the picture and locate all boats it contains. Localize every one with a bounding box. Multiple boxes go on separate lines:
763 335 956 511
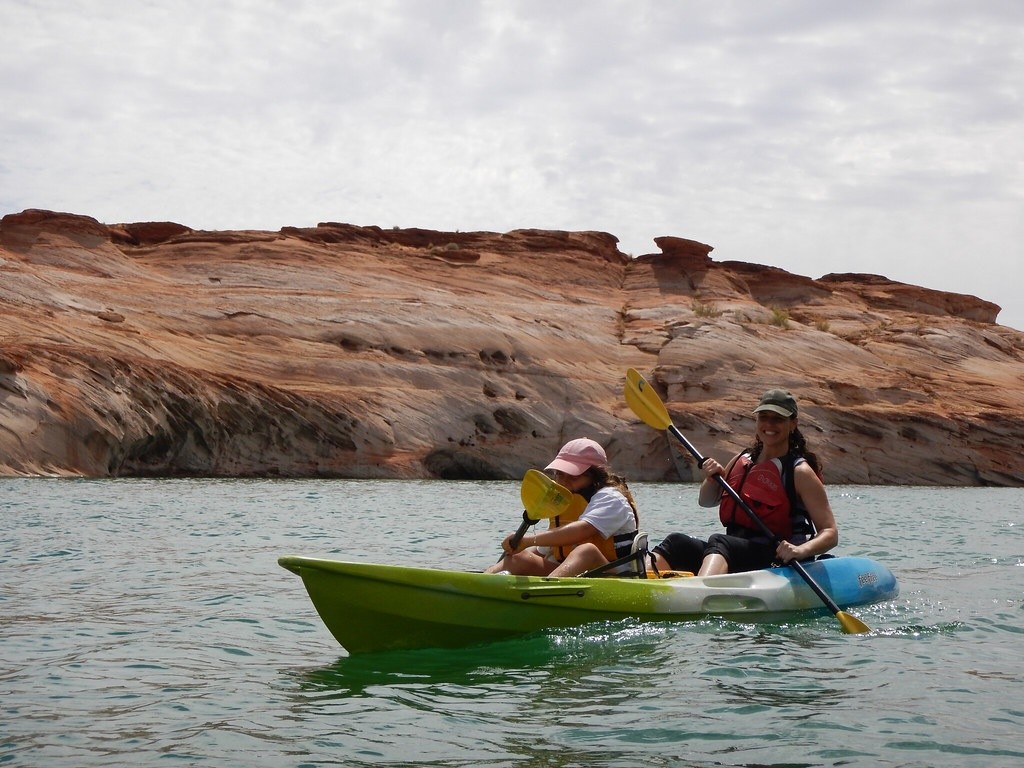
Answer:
277 554 899 656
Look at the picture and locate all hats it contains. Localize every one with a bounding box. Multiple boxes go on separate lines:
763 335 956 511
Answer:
543 436 607 476
752 388 798 417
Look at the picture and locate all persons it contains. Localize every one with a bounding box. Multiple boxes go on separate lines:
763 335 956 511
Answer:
647 389 838 580
484 437 638 576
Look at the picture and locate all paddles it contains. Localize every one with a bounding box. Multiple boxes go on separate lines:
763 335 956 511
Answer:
622 367 875 636
497 468 576 564
